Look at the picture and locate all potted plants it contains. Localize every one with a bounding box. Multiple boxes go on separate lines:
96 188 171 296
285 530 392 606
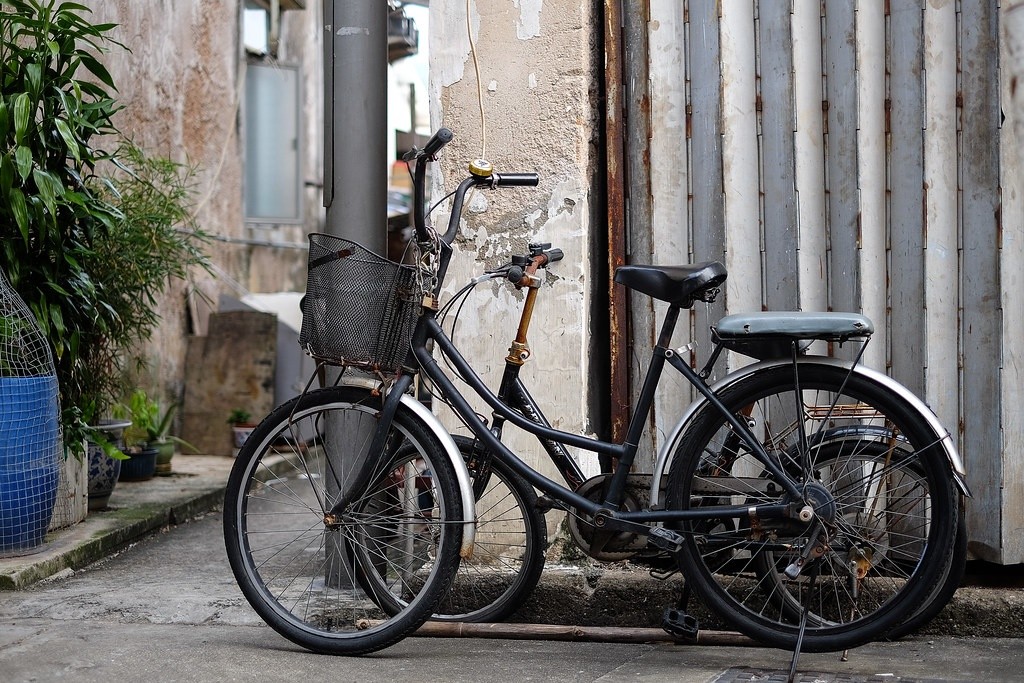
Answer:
228 410 260 449
0 0 227 560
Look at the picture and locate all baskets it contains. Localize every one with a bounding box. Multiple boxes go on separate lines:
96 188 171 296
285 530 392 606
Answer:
297 233 432 373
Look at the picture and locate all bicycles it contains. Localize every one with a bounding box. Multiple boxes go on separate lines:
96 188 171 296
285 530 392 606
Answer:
223 127 972 683
